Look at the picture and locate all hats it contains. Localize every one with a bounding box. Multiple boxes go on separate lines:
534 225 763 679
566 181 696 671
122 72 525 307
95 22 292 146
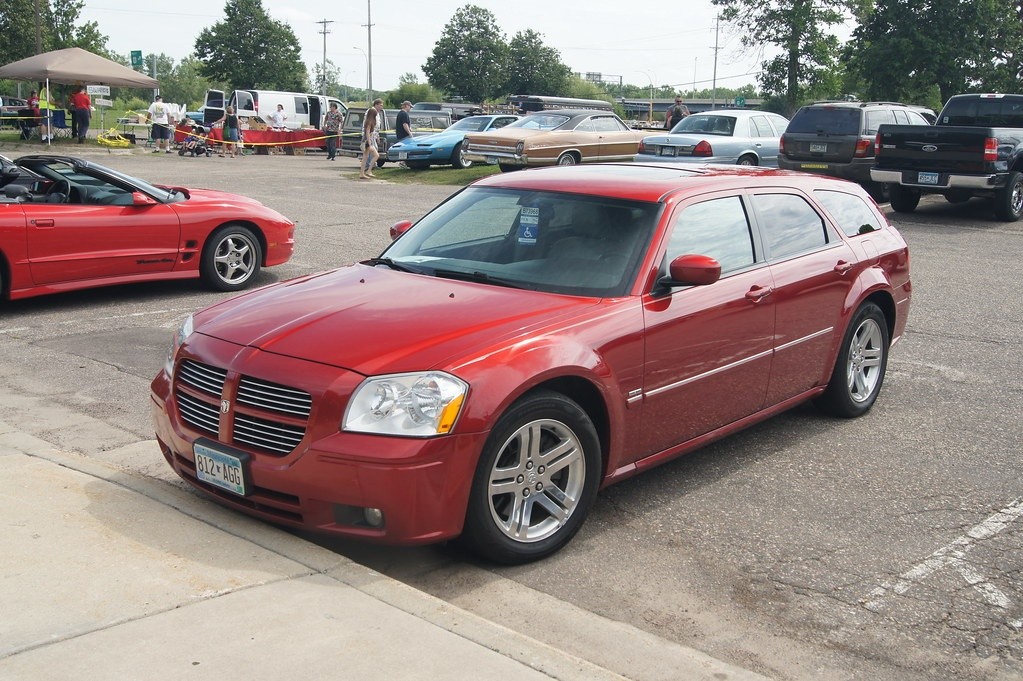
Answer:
401 100 414 107
155 95 163 100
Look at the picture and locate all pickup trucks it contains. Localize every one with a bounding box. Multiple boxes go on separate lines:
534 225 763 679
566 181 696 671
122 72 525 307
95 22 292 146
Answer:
868 93 1023 222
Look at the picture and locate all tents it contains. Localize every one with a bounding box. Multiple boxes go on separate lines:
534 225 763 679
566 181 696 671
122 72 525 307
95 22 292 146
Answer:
0 47 159 95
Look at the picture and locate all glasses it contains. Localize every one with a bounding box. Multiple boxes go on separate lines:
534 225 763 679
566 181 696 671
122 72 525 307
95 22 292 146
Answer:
676 101 682 103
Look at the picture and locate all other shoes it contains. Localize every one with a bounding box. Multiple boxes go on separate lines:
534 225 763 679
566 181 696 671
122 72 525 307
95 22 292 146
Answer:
219 154 225 157
230 154 235 157
152 150 159 153
166 148 174 153
79 135 84 143
332 156 335 161
366 171 375 177
360 174 370 179
327 155 333 160
399 162 410 169
42 137 55 143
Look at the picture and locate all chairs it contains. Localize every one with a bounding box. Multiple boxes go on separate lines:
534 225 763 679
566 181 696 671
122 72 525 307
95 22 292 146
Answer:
4 184 34 202
544 203 625 261
39 107 72 138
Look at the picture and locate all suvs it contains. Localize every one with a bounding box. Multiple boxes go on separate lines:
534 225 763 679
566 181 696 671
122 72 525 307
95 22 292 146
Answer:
776 98 933 203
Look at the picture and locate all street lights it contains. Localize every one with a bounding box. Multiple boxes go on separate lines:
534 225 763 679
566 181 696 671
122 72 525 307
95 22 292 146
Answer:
354 46 368 90
691 56 698 99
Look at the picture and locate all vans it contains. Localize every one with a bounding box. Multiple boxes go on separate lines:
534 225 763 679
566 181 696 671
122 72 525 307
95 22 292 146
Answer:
340 107 452 168
203 88 348 156
411 102 488 128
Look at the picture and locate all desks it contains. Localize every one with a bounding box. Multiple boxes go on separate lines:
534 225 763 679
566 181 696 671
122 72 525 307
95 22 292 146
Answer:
174 126 342 154
117 122 154 148
0 115 48 142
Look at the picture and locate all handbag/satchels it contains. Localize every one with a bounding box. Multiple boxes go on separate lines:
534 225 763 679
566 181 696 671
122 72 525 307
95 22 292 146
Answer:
223 126 230 139
237 140 243 148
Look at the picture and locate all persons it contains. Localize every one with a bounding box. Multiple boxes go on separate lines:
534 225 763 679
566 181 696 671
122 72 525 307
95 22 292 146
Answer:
27 90 40 110
396 101 414 169
68 84 92 144
145 95 206 154
323 103 342 161
0 96 3 112
268 104 287 131
359 99 384 179
212 106 245 158
38 82 62 143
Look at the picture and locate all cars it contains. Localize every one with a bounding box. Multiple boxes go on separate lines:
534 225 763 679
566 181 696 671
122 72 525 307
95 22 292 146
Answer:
151 164 913 568
185 105 205 125
458 109 669 172
0 154 295 313
0 95 32 111
387 115 555 169
632 109 791 170
